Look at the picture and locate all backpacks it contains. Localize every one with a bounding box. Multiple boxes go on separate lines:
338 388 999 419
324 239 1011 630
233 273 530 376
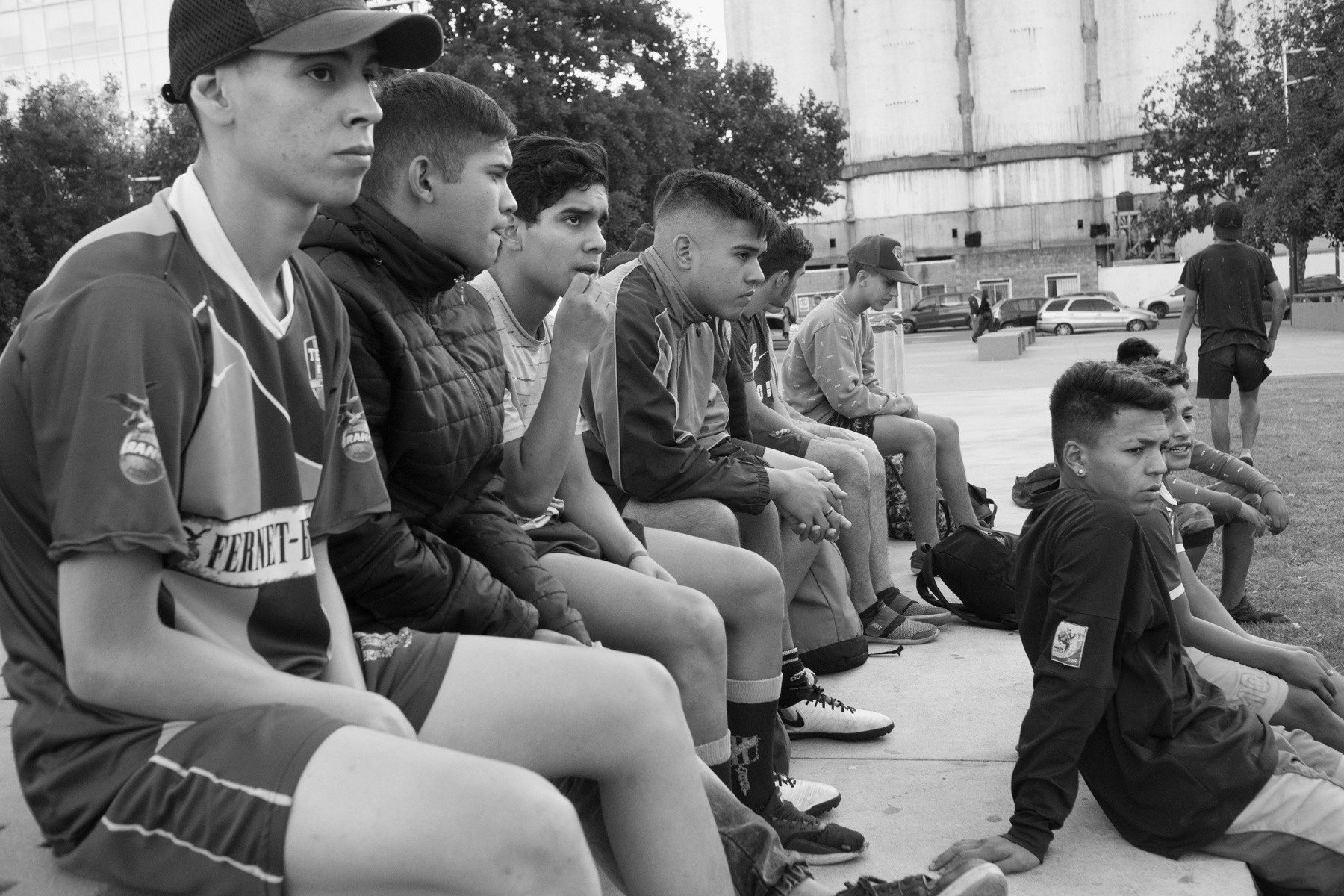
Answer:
881 451 957 542
915 521 1021 631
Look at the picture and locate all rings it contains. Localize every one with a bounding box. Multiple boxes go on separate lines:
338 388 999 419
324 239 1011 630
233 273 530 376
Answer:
824 507 833 516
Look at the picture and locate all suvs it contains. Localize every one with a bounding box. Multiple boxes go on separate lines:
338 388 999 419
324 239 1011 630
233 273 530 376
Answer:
989 292 1159 337
1284 275 1344 303
903 292 982 334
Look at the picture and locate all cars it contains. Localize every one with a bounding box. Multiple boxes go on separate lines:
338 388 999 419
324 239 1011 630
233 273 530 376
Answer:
1139 284 1292 327
764 314 788 341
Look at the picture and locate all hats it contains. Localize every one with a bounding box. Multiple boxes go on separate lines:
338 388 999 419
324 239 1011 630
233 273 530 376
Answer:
159 0 441 104
846 233 918 287
1213 202 1244 240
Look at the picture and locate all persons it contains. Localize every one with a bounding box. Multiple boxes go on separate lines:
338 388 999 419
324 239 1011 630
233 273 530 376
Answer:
1117 338 1160 366
927 360 1344 896
730 218 952 645
781 234 1020 577
0 0 733 896
579 171 895 815
782 306 791 338
1162 439 1292 622
599 170 834 608
970 289 997 344
1129 354 1344 751
788 317 800 341
968 287 981 335
813 294 822 305
298 71 1008 896
1171 201 1286 466
460 133 869 863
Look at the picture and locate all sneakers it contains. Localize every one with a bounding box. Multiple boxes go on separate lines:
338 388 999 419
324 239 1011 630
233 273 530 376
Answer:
860 600 940 645
761 786 868 865
776 685 894 742
771 767 841 817
888 591 951 626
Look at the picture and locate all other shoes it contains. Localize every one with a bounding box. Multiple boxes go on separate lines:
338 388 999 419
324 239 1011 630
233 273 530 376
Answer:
910 549 927 575
834 856 1007 896
1238 448 1255 468
1225 590 1291 625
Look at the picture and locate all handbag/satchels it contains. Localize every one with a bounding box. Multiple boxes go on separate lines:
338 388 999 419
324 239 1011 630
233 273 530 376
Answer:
966 482 997 529
